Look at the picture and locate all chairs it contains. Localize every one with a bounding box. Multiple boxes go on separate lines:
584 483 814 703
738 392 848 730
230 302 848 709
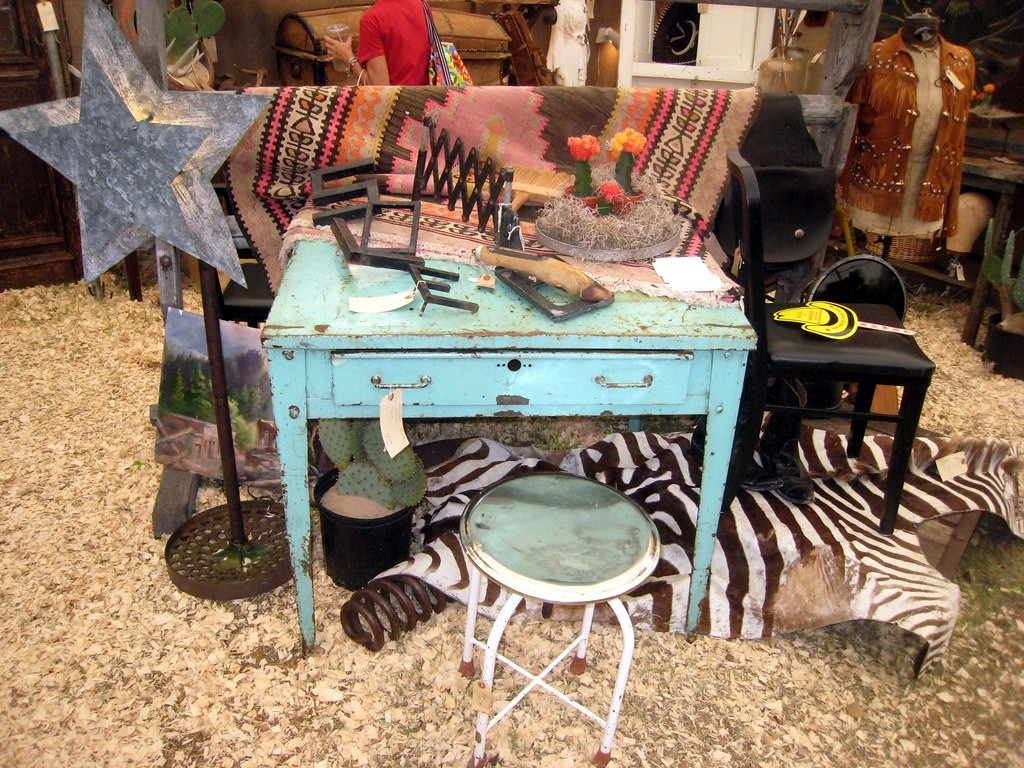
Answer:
724 144 936 536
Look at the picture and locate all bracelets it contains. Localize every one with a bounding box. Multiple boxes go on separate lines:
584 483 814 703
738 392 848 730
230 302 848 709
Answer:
345 57 358 77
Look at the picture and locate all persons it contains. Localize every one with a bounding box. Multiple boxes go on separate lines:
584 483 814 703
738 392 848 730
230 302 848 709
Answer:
324 0 434 86
840 12 975 239
546 0 589 86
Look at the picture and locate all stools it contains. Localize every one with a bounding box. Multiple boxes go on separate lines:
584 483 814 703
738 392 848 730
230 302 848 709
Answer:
459 471 661 768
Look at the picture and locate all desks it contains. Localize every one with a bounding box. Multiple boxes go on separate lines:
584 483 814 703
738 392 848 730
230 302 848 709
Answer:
260 237 757 660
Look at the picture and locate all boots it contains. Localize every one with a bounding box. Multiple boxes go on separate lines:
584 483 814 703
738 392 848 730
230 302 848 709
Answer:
691 361 784 490
758 373 815 503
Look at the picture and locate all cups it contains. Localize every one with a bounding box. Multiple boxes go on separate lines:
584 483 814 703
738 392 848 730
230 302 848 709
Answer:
325 23 351 72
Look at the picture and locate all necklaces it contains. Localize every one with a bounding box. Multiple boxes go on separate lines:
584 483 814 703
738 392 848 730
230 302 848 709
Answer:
910 39 941 58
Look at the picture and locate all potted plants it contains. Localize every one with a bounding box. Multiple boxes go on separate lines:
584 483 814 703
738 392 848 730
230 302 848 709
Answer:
981 218 1024 380
562 135 602 217
607 128 647 217
312 417 428 592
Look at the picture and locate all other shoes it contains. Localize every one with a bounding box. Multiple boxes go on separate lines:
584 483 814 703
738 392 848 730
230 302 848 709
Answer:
804 381 841 417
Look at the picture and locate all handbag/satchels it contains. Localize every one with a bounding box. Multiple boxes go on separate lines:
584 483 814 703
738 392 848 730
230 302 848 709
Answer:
423 4 473 86
